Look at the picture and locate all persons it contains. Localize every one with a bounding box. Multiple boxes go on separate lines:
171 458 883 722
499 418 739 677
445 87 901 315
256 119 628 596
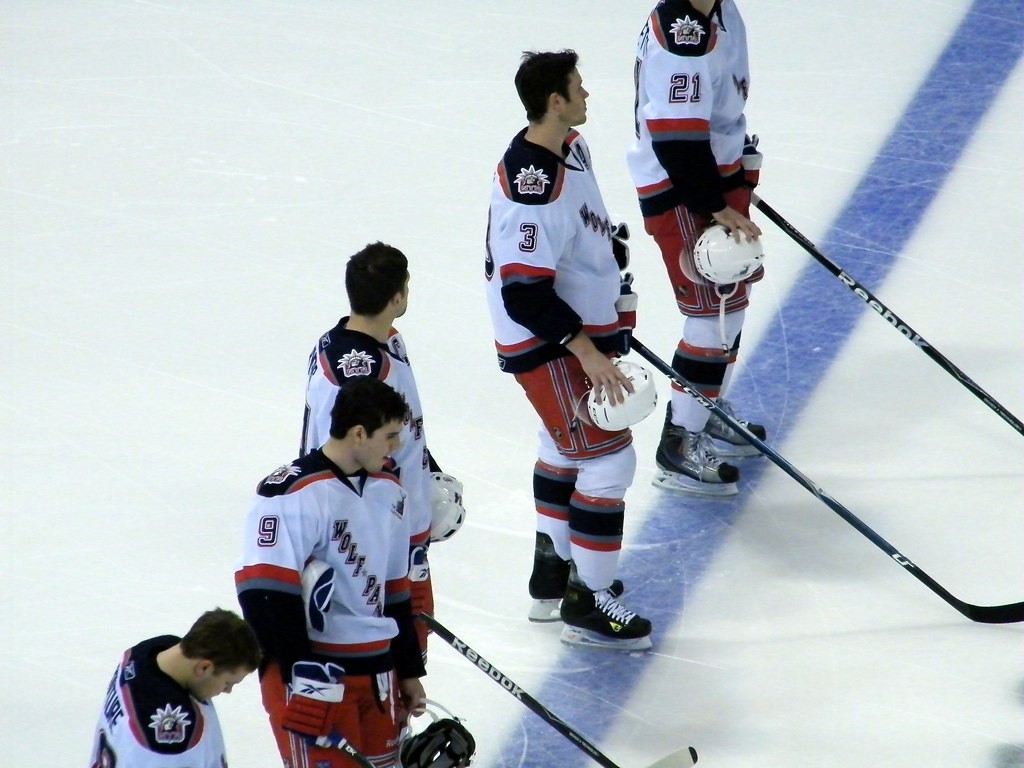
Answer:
297 242 466 666
234 376 426 768
485 52 652 638
626 0 767 484
89 608 263 768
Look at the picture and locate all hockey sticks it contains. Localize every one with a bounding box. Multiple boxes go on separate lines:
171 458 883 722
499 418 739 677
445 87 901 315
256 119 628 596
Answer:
415 610 702 768
628 335 1024 627
749 189 1024 439
330 729 378 768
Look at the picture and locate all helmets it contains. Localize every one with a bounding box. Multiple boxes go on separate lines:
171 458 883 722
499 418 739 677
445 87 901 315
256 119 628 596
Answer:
693 223 763 284
427 471 466 543
587 356 657 431
394 718 475 768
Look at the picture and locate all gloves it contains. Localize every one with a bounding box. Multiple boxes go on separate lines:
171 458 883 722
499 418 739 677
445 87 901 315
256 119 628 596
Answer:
279 661 347 748
299 554 334 631
615 290 638 330
742 134 763 191
611 223 630 270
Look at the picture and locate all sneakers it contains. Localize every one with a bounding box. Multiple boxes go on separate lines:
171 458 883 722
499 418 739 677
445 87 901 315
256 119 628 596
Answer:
699 398 766 457
652 399 741 496
560 557 652 650
528 530 625 623
